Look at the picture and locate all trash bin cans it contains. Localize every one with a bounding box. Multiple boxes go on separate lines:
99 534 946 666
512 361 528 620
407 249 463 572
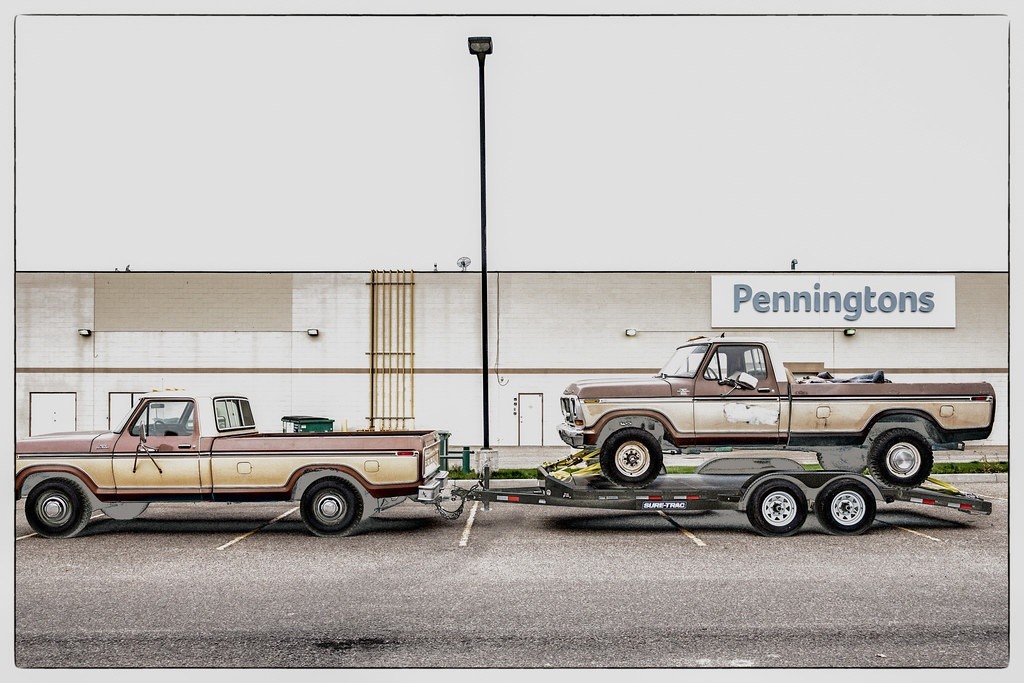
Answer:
439 431 451 472
281 416 335 433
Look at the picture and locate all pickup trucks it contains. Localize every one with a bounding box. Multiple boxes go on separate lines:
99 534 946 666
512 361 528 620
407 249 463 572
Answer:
16 391 449 538
556 336 996 489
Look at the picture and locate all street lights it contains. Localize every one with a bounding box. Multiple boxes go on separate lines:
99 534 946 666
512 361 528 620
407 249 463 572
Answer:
469 37 495 448
